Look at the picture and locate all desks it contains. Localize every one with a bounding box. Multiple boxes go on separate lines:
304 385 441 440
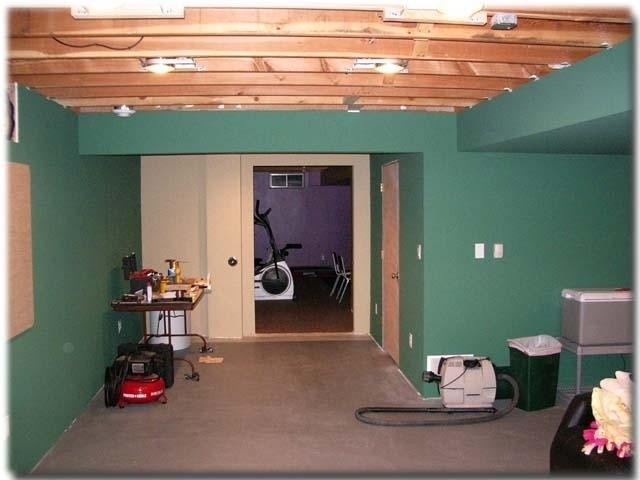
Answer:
556 338 633 405
112 287 211 382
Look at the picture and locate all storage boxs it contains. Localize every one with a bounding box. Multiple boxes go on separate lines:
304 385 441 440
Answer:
562 288 633 345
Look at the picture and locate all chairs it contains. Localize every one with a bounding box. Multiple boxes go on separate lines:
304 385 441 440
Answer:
549 389 635 480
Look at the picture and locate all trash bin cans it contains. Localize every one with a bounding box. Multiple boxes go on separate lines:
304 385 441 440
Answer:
507 334 563 411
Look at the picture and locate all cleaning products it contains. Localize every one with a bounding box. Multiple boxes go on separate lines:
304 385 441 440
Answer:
174 261 189 283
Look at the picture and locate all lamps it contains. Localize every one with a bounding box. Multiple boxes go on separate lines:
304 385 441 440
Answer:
142 57 175 75
113 105 134 117
490 15 518 30
376 59 405 75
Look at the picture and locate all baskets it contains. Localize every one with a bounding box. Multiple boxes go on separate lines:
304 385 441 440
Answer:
116 343 175 388
